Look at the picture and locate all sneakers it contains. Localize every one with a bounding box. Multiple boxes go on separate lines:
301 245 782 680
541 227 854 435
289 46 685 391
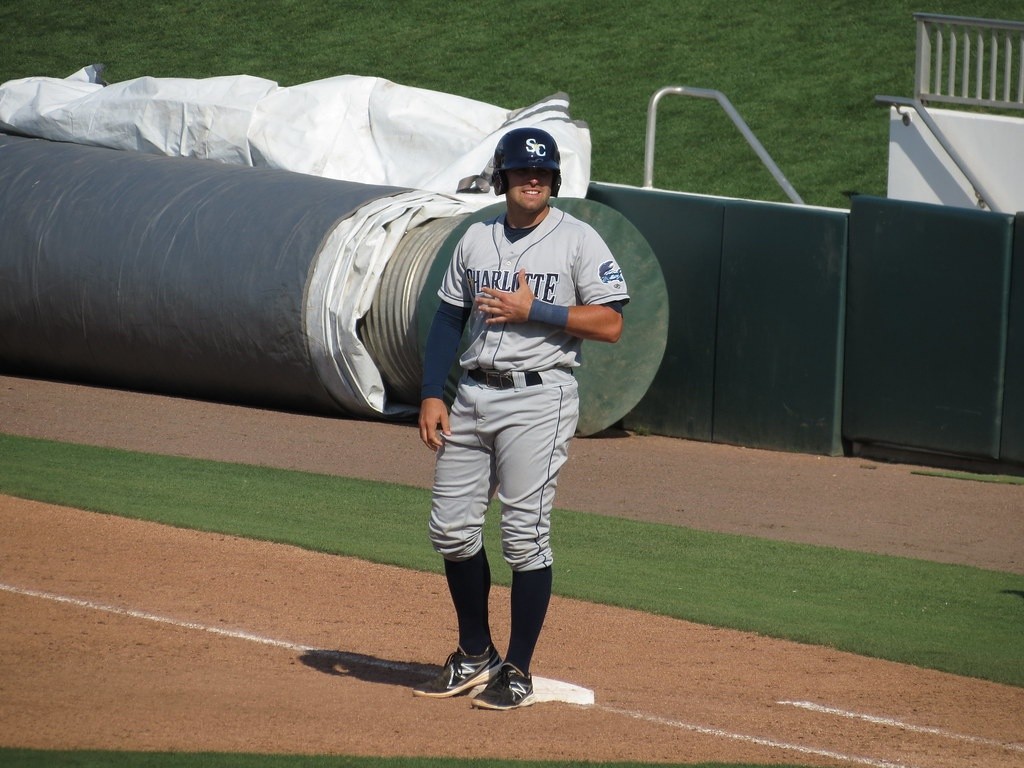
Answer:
412 643 504 698
471 663 535 710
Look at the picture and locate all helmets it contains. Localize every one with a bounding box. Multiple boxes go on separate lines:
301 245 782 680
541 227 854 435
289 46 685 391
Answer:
494 128 561 199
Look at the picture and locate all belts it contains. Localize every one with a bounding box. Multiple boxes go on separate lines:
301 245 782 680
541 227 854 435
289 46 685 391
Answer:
468 367 573 389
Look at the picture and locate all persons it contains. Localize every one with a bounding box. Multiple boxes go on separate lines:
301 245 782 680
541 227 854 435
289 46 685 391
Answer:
412 128 631 709
